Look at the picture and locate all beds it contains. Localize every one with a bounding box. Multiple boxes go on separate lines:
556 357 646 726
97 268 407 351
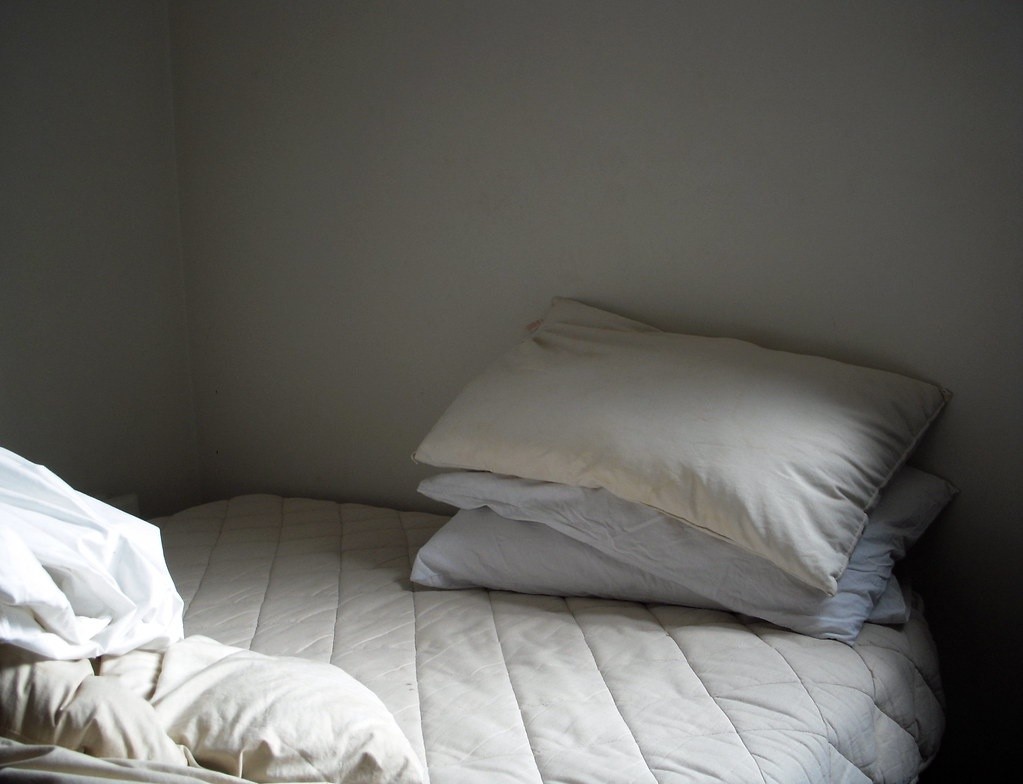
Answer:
0 496 963 784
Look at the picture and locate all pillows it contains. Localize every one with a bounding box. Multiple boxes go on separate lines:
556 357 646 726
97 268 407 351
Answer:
405 510 915 627
413 297 952 597
413 467 962 651
